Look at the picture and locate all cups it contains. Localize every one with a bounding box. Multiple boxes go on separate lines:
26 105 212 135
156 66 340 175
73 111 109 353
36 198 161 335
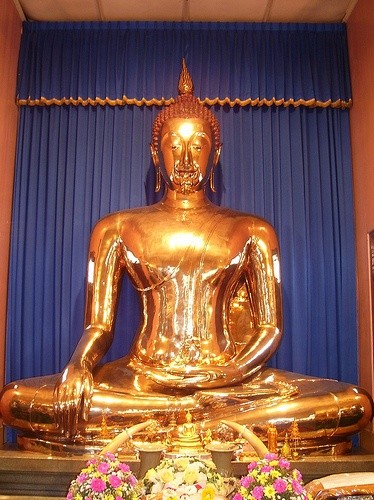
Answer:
137 448 162 482
210 448 233 479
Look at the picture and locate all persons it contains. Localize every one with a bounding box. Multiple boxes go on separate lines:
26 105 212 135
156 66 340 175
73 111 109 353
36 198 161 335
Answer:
0 57 374 459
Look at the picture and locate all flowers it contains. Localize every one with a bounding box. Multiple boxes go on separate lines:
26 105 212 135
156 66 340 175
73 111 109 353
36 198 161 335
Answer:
66 452 142 500
138 455 241 500
228 453 314 500
